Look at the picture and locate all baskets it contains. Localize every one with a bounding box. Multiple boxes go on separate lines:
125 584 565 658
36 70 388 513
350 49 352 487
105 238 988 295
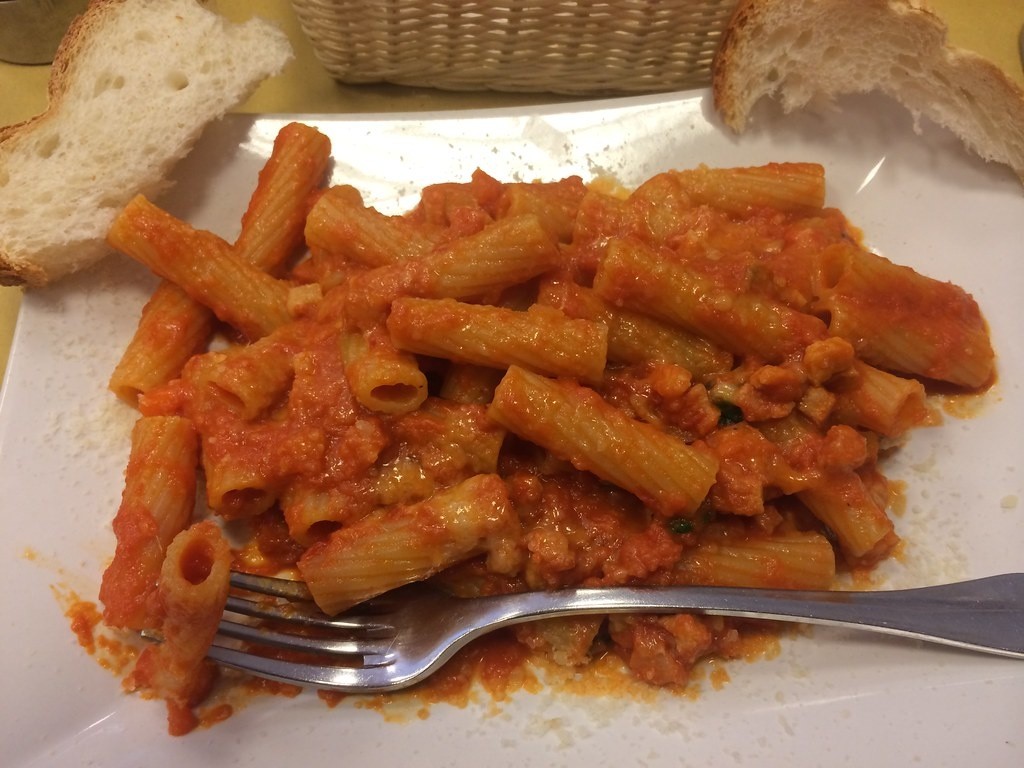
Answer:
290 1 745 91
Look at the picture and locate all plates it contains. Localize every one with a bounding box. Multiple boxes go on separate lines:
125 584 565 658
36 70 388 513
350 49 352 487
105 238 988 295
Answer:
2 85 1023 768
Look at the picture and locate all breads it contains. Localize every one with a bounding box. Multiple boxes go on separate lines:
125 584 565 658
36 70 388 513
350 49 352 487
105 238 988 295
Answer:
0 0 294 285
711 0 1024 180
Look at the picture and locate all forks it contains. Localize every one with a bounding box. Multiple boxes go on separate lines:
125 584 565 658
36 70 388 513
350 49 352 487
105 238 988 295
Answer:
139 570 1023 692
